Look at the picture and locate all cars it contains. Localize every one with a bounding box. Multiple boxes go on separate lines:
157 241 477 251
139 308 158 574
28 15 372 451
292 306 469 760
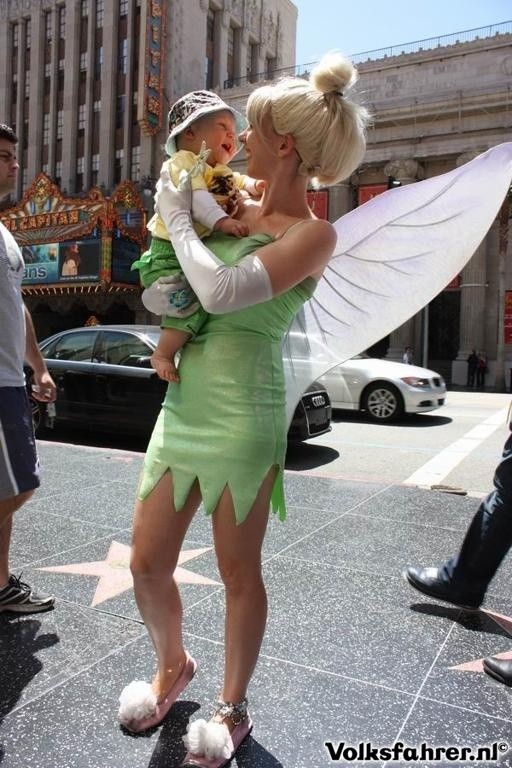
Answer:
277 331 447 424
17 324 335 445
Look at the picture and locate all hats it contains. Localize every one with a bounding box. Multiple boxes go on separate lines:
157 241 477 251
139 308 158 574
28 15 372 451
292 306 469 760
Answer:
165 90 249 160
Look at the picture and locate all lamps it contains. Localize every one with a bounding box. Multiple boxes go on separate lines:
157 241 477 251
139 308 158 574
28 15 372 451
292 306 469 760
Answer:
144 176 158 196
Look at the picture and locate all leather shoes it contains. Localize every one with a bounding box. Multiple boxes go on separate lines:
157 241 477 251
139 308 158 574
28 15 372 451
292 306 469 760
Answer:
405 566 484 610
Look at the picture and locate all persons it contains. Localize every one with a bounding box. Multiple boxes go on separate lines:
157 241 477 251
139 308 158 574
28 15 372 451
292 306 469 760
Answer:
128 88 265 384
402 346 416 366
84 315 97 327
476 348 488 388
61 244 82 277
465 348 481 387
115 50 371 767
403 416 512 611
0 123 58 616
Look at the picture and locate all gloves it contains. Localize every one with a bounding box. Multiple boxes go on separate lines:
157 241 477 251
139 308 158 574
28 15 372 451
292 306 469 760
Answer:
142 272 201 318
154 158 273 318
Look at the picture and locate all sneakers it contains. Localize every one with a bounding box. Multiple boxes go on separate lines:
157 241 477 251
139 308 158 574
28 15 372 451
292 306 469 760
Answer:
182 700 253 768
118 649 197 733
0 571 56 613
